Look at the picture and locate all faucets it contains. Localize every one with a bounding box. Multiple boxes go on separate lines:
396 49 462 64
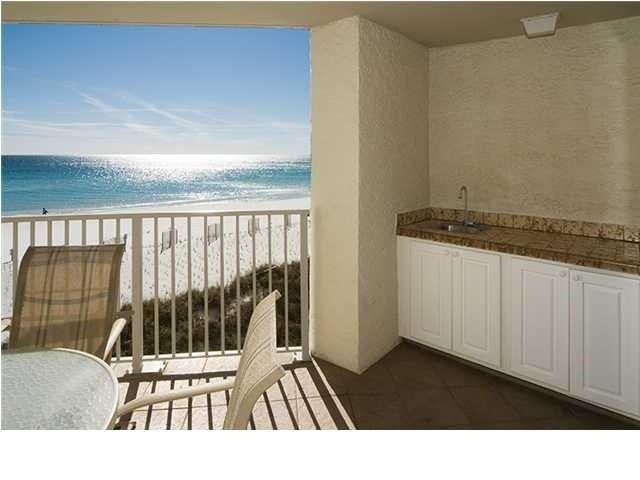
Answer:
457 185 468 225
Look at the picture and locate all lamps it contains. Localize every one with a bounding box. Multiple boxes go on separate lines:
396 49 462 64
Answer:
520 10 560 38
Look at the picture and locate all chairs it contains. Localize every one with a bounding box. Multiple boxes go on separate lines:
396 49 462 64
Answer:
1 245 128 370
106 290 287 430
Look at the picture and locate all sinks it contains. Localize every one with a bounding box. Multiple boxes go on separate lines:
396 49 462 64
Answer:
432 223 493 234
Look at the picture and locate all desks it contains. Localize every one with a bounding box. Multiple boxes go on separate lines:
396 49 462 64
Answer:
1 346 119 431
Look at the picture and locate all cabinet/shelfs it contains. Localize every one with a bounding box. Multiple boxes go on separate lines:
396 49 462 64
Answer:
408 240 502 368
510 259 640 420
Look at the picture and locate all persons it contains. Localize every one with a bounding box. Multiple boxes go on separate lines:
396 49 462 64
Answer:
42 208 48 215
